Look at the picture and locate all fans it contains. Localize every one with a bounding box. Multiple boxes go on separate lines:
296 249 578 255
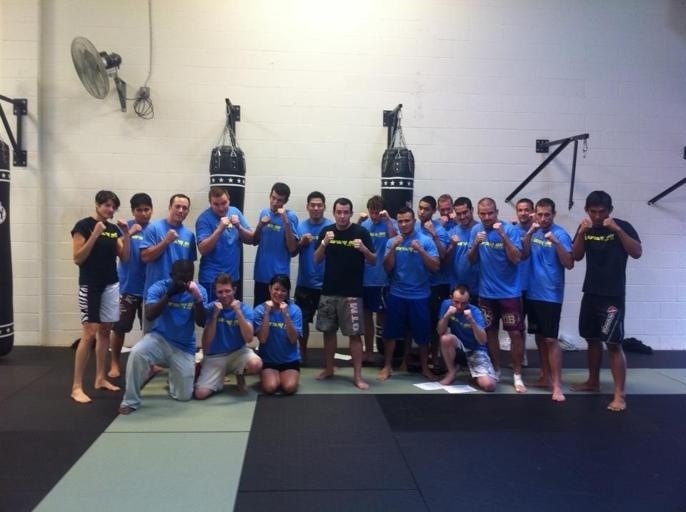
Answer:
67 34 131 116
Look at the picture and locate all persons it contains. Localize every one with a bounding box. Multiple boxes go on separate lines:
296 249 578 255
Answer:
355 195 403 363
312 196 378 390
67 188 131 404
194 186 254 310
252 182 300 311
377 205 441 382
138 193 197 338
193 271 263 401
569 190 644 411
413 193 575 403
118 259 209 415
251 274 305 395
107 191 153 380
294 191 336 367
